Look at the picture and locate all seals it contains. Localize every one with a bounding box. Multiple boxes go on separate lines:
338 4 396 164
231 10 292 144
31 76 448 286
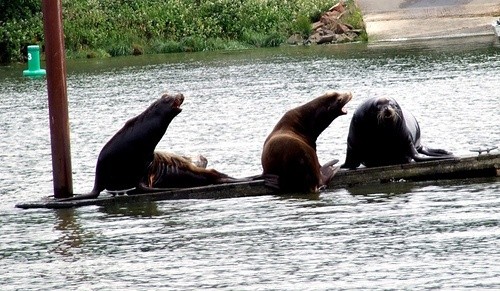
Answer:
339 94 460 168
220 91 352 195
55 93 237 202
